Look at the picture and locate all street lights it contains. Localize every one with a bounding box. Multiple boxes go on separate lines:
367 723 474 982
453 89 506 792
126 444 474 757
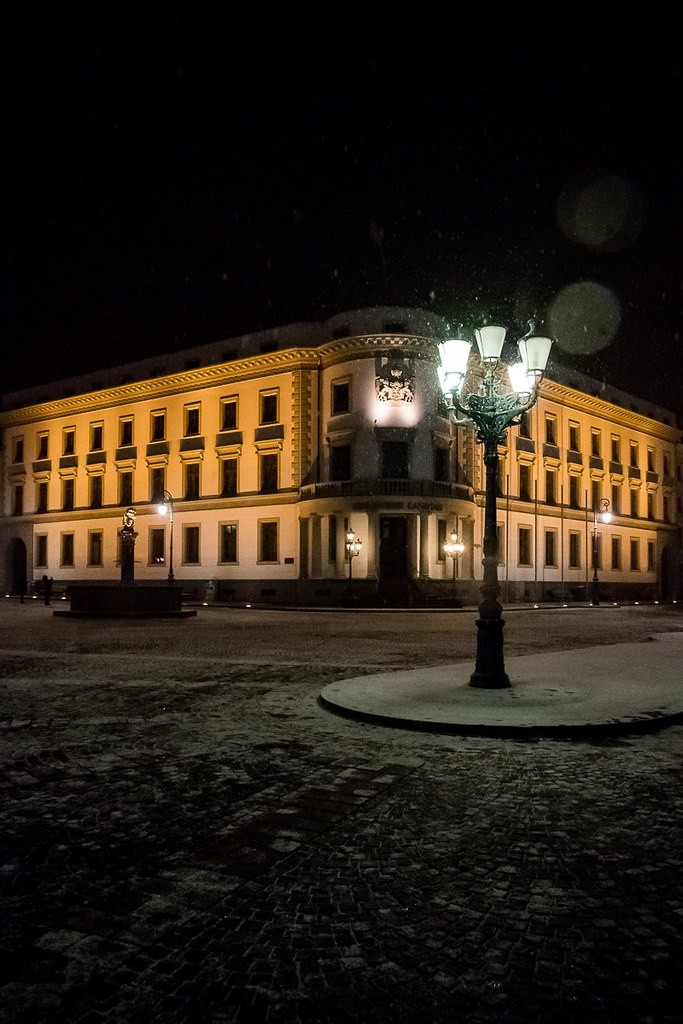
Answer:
345 528 362 602
160 490 175 586
591 497 613 605
445 531 465 600
433 291 549 685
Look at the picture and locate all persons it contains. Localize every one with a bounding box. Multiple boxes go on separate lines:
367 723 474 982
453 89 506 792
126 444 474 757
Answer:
43 575 54 606
